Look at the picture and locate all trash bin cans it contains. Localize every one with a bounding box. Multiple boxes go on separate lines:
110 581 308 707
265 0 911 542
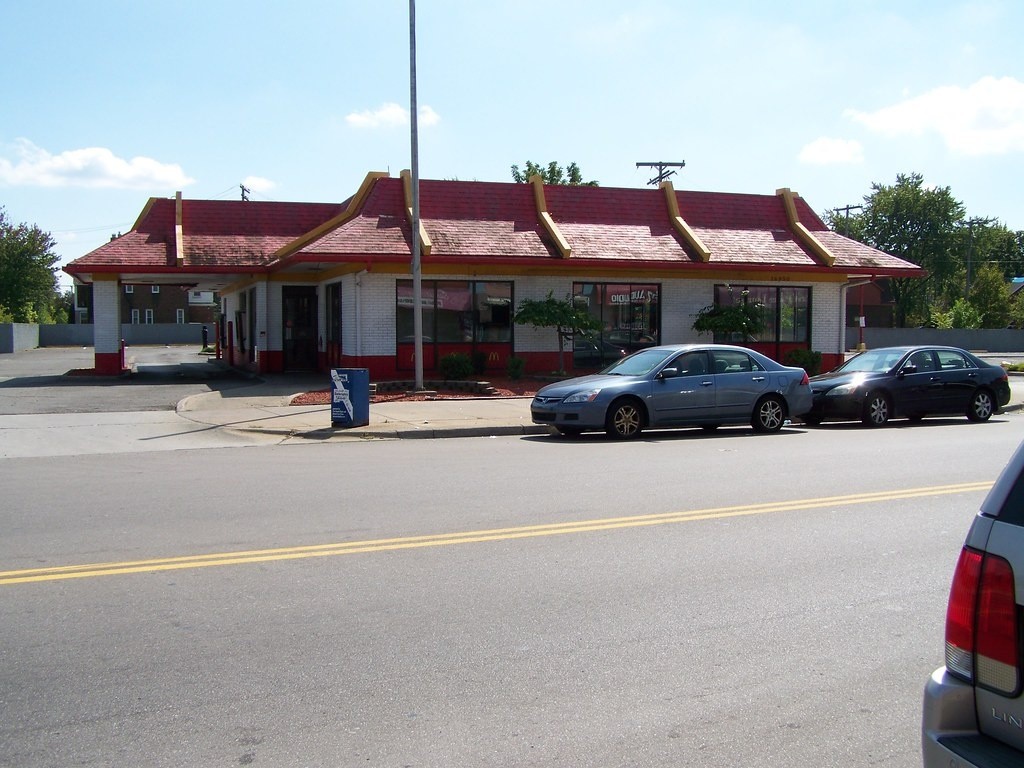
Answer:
331 368 370 427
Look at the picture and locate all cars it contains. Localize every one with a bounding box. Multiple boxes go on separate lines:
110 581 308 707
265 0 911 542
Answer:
532 343 814 441
798 346 1013 428
